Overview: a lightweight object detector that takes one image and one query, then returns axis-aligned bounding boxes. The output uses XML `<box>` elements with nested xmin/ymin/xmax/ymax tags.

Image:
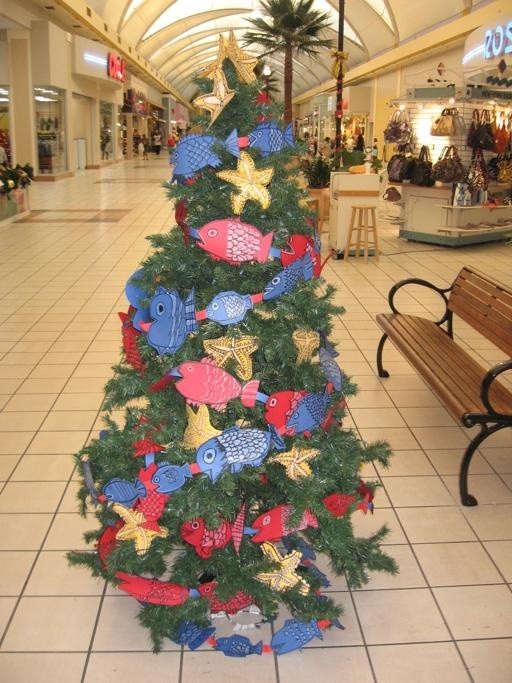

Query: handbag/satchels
<box><xmin>383</xmin><ymin>108</ymin><xmax>512</xmax><ymax>206</ymax></box>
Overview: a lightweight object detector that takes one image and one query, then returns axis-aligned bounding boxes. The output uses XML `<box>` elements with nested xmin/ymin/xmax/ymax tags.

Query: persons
<box><xmin>366</xmin><ymin>145</ymin><xmax>378</xmax><ymax>160</ymax></box>
<box><xmin>172</xmin><ymin>126</ymin><xmax>182</xmax><ymax>141</ymax></box>
<box><xmin>323</xmin><ymin>137</ymin><xmax>331</xmax><ymax>148</ymax></box>
<box><xmin>356</xmin><ymin>134</ymin><xmax>365</xmax><ymax>153</ymax></box>
<box><xmin>138</xmin><ymin>134</ymin><xmax>148</xmax><ymax>160</ymax></box>
<box><xmin>167</xmin><ymin>135</ymin><xmax>174</xmax><ymax>155</ymax></box>
<box><xmin>100</xmin><ymin>131</ymin><xmax>110</xmax><ymax>160</ymax></box>
<box><xmin>153</xmin><ymin>130</ymin><xmax>162</xmax><ymax>155</ymax></box>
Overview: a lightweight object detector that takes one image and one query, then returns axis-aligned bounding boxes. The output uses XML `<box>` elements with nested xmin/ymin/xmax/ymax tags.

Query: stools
<box><xmin>342</xmin><ymin>202</ymin><xmax>380</xmax><ymax>265</ymax></box>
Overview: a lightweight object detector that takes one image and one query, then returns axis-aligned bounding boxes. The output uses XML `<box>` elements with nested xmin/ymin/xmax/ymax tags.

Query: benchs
<box><xmin>374</xmin><ymin>265</ymin><xmax>512</xmax><ymax>507</ymax></box>
